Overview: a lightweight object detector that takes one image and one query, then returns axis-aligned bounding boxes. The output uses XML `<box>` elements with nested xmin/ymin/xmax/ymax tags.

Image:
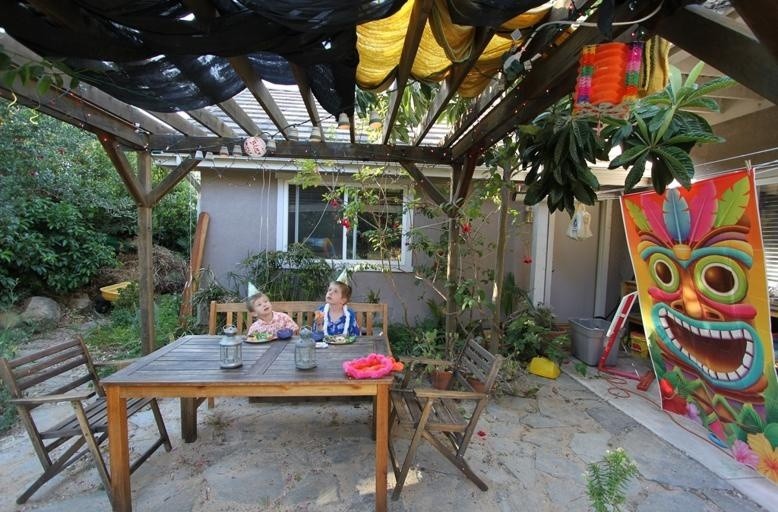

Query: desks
<box><xmin>98</xmin><ymin>335</ymin><xmax>393</xmax><ymax>511</ymax></box>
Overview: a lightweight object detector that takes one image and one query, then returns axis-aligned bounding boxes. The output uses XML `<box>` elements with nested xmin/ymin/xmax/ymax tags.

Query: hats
<box><xmin>335</xmin><ymin>267</ymin><xmax>348</xmax><ymax>286</ymax></box>
<box><xmin>247</xmin><ymin>282</ymin><xmax>259</xmax><ymax>297</ymax></box>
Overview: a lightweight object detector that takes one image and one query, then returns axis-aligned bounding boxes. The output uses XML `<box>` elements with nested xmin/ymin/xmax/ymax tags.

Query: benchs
<box><xmin>387</xmin><ymin>335</ymin><xmax>503</xmax><ymax>501</ymax></box>
<box><xmin>208</xmin><ymin>300</ymin><xmax>394</xmax><ymax>410</ymax></box>
<box><xmin>0</xmin><ymin>333</ymin><xmax>172</xmax><ymax>512</ymax></box>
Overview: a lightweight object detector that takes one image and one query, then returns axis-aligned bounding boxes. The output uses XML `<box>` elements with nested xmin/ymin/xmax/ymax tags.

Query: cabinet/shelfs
<box><xmin>619</xmin><ymin>280</ymin><xmax>643</xmax><ymax>337</ymax></box>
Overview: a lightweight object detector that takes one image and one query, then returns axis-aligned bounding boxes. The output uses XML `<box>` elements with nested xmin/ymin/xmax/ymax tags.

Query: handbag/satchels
<box><xmin>431</xmin><ymin>370</ymin><xmax>452</xmax><ymax>390</ymax></box>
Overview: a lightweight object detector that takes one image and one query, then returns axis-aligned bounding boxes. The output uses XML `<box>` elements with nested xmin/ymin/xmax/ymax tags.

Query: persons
<box><xmin>311</xmin><ymin>268</ymin><xmax>361</xmax><ymax>336</ymax></box>
<box><xmin>246</xmin><ymin>281</ymin><xmax>299</xmax><ymax>336</ymax></box>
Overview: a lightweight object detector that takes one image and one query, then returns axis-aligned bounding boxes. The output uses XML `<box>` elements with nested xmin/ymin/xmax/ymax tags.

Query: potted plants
<box><xmin>412</xmin><ymin>329</ymin><xmax>458</xmax><ymax>389</ymax></box>
<box><xmin>517</xmin><ymin>287</ymin><xmax>567</xmax><ymax>356</ymax></box>
<box><xmin>467</xmin><ymin>336</ymin><xmax>487</xmax><ymax>394</ymax></box>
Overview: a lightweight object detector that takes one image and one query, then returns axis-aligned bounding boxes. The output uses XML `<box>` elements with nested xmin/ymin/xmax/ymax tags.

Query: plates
<box><xmin>323</xmin><ymin>335</ymin><xmax>356</xmax><ymax>344</ymax></box>
<box><xmin>244</xmin><ymin>337</ymin><xmax>277</xmax><ymax>343</ymax></box>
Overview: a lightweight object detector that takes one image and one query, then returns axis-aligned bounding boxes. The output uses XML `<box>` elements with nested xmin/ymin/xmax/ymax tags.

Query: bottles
<box><xmin>294</xmin><ymin>325</ymin><xmax>316</xmax><ymax>369</ymax></box>
<box><xmin>219</xmin><ymin>324</ymin><xmax>242</xmax><ymax>369</ymax></box>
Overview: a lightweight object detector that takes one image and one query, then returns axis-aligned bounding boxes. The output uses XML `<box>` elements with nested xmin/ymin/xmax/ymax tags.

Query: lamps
<box><xmin>194</xmin><ymin>90</ymin><xmax>384</xmax><ymax>161</ymax></box>
